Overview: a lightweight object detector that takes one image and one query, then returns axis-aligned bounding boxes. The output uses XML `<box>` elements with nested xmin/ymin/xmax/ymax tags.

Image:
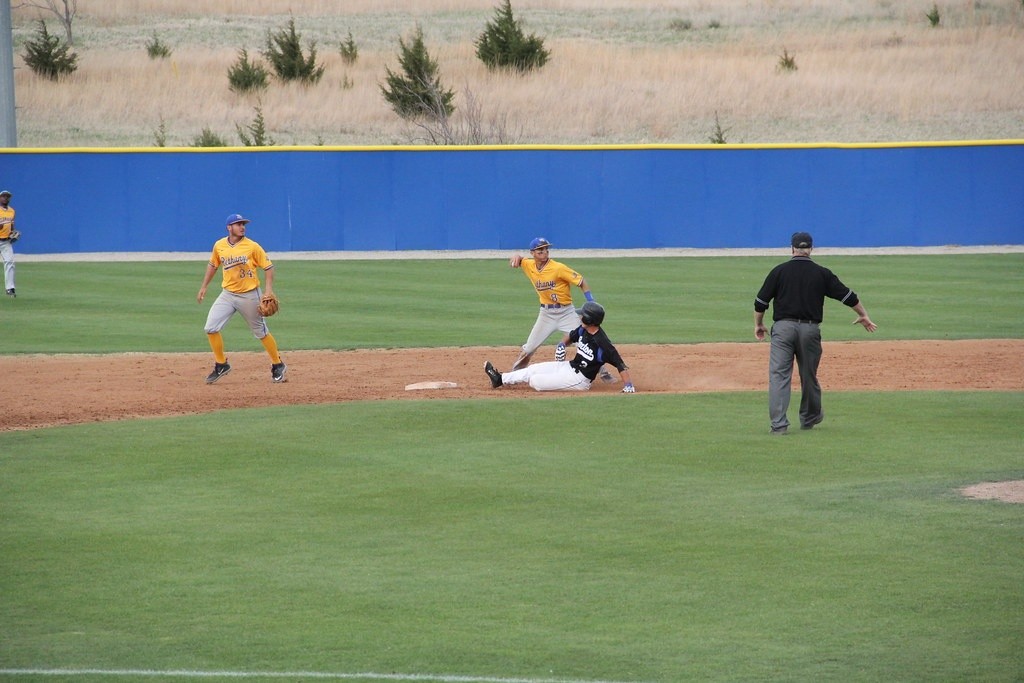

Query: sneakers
<box><xmin>601</xmin><ymin>371</ymin><xmax>617</xmax><ymax>383</ymax></box>
<box><xmin>205</xmin><ymin>357</ymin><xmax>231</xmax><ymax>384</ymax></box>
<box><xmin>6</xmin><ymin>288</ymin><xmax>16</xmax><ymax>298</ymax></box>
<box><xmin>484</xmin><ymin>360</ymin><xmax>502</xmax><ymax>389</ymax></box>
<box><xmin>271</xmin><ymin>357</ymin><xmax>287</xmax><ymax>383</ymax></box>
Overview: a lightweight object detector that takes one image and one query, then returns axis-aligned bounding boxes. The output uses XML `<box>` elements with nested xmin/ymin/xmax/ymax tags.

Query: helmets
<box><xmin>573</xmin><ymin>302</ymin><xmax>605</xmax><ymax>325</ymax></box>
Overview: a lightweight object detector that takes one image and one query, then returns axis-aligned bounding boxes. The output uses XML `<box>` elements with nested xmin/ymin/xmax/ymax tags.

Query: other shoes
<box><xmin>769</xmin><ymin>428</ymin><xmax>787</xmax><ymax>436</ymax></box>
<box><xmin>800</xmin><ymin>409</ymin><xmax>824</xmax><ymax>429</ymax></box>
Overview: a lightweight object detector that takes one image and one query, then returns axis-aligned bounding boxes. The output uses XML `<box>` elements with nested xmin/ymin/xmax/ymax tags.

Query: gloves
<box><xmin>555</xmin><ymin>343</ymin><xmax>566</xmax><ymax>362</ymax></box>
<box><xmin>620</xmin><ymin>383</ymin><xmax>635</xmax><ymax>394</ymax></box>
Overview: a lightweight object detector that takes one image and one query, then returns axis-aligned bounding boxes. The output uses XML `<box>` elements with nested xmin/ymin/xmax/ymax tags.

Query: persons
<box><xmin>509</xmin><ymin>237</ymin><xmax>619</xmax><ymax>384</ymax></box>
<box><xmin>753</xmin><ymin>231</ymin><xmax>878</xmax><ymax>435</ymax></box>
<box><xmin>483</xmin><ymin>301</ymin><xmax>635</xmax><ymax>393</ymax></box>
<box><xmin>197</xmin><ymin>214</ymin><xmax>287</xmax><ymax>385</ymax></box>
<box><xmin>0</xmin><ymin>190</ymin><xmax>21</xmax><ymax>298</ymax></box>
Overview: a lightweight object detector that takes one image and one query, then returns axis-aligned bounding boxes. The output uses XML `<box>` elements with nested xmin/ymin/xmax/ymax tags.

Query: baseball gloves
<box><xmin>257</xmin><ymin>293</ymin><xmax>279</xmax><ymax>317</ymax></box>
<box><xmin>9</xmin><ymin>231</ymin><xmax>21</xmax><ymax>244</ymax></box>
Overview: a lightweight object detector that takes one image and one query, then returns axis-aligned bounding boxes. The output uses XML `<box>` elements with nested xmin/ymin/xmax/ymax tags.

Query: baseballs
<box><xmin>512</xmin><ymin>261</ymin><xmax>515</xmax><ymax>267</ymax></box>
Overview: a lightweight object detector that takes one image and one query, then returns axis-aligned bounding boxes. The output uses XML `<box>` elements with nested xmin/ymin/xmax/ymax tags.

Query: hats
<box><xmin>226</xmin><ymin>214</ymin><xmax>250</xmax><ymax>225</ymax></box>
<box><xmin>529</xmin><ymin>238</ymin><xmax>553</xmax><ymax>250</ymax></box>
<box><xmin>0</xmin><ymin>190</ymin><xmax>12</xmax><ymax>196</ymax></box>
<box><xmin>792</xmin><ymin>231</ymin><xmax>813</xmax><ymax>249</ymax></box>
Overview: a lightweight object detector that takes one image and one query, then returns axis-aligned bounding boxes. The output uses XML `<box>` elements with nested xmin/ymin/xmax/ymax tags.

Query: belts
<box><xmin>779</xmin><ymin>318</ymin><xmax>816</xmax><ymax>324</ymax></box>
<box><xmin>541</xmin><ymin>304</ymin><xmax>565</xmax><ymax>308</ymax></box>
<box><xmin>570</xmin><ymin>364</ymin><xmax>593</xmax><ymax>384</ymax></box>
